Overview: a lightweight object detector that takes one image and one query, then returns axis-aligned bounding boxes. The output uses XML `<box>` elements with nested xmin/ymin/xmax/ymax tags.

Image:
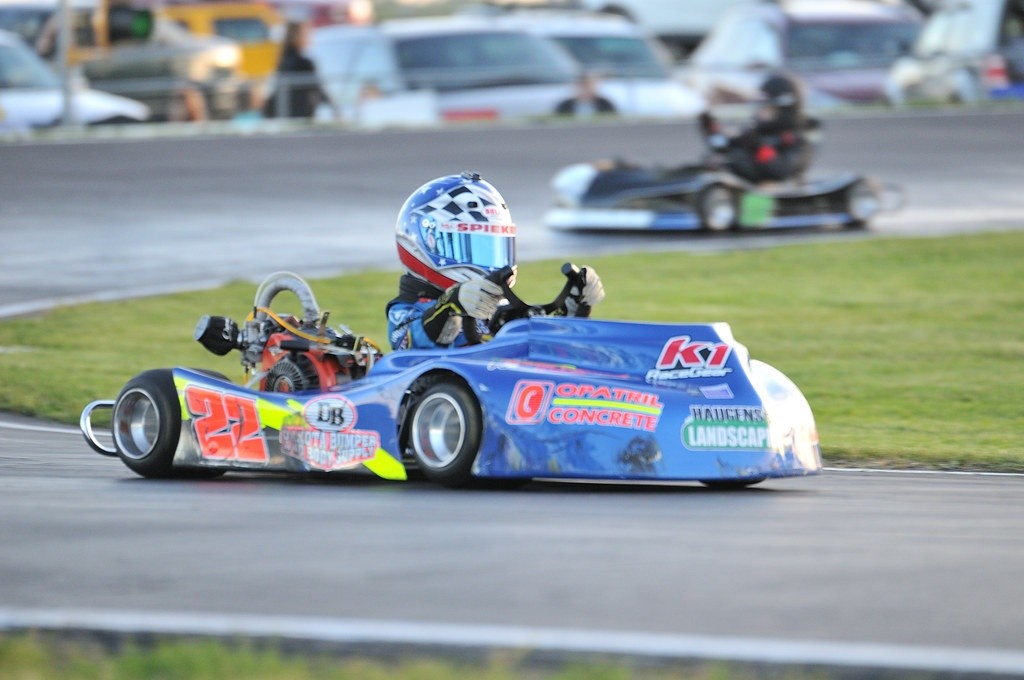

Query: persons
<box><xmin>274</xmin><ymin>22</ymin><xmax>336</xmax><ymax>118</ymax></box>
<box><xmin>386</xmin><ymin>171</ymin><xmax>604</xmax><ymax>350</ymax></box>
<box><xmin>554</xmin><ymin>69</ymin><xmax>618</xmax><ymax>116</ymax></box>
<box><xmin>160</xmin><ymin>84</ymin><xmax>204</xmax><ymax>121</ymax></box>
<box><xmin>699</xmin><ymin>77</ymin><xmax>814</xmax><ymax>183</ymax></box>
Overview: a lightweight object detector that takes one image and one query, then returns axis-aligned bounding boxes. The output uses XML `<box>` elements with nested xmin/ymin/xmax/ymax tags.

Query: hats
<box><xmin>757</xmin><ymin>76</ymin><xmax>802</xmax><ymax>131</ymax></box>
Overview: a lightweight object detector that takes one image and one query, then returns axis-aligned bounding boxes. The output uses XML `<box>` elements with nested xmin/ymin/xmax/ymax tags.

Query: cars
<box><xmin>0</xmin><ymin>0</ymin><xmax>375</xmax><ymax>132</ymax></box>
<box><xmin>684</xmin><ymin>2</ymin><xmax>930</xmax><ymax>104</ymax></box>
<box><xmin>285</xmin><ymin>0</ymin><xmax>756</xmax><ymax>124</ymax></box>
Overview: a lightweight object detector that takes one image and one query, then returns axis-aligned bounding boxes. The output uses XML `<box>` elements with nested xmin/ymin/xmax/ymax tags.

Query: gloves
<box><xmin>554</xmin><ymin>265</ymin><xmax>605</xmax><ymax>319</ymax></box>
<box><xmin>447</xmin><ymin>277</ymin><xmax>503</xmax><ymax>320</ymax></box>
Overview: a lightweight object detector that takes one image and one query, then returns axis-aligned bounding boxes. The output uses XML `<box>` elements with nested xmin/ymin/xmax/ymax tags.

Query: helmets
<box><xmin>394</xmin><ymin>170</ymin><xmax>518</xmax><ymax>294</ymax></box>
